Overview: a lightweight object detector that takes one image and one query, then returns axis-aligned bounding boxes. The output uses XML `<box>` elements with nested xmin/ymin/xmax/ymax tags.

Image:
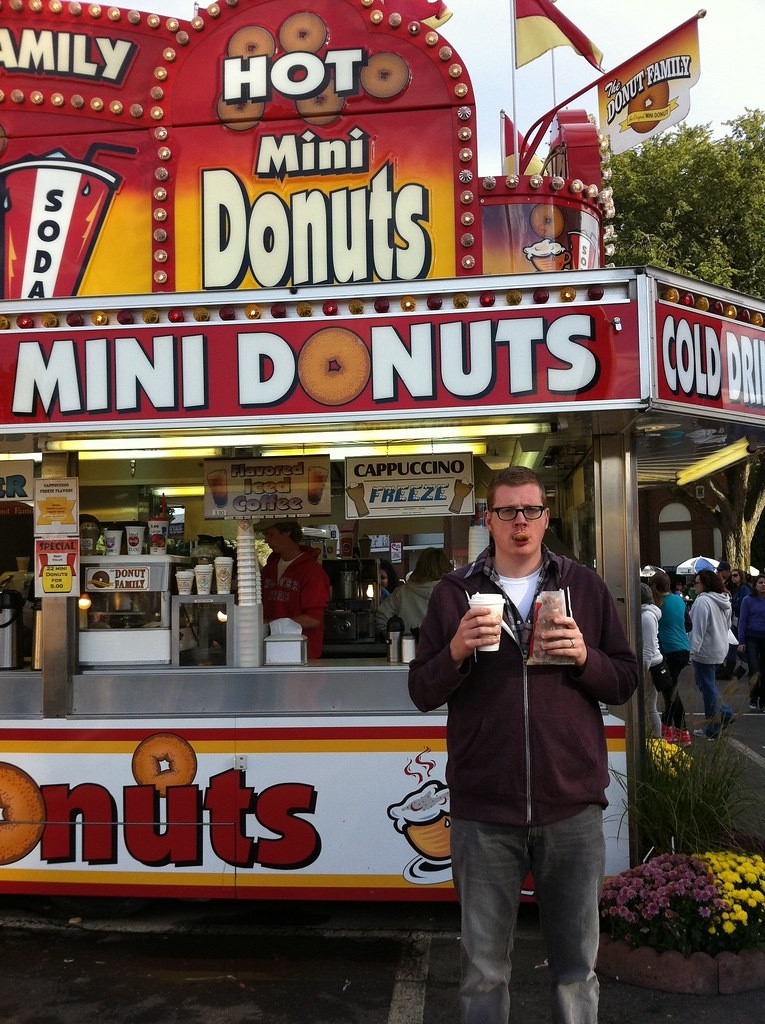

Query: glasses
<box><xmin>731</xmin><ymin>574</ymin><xmax>738</xmax><ymax>578</ymax></box>
<box><xmin>694</xmin><ymin>582</ymin><xmax>701</xmax><ymax>584</ymax></box>
<box><xmin>490</xmin><ymin>505</ymin><xmax>546</xmax><ymax>520</ymax></box>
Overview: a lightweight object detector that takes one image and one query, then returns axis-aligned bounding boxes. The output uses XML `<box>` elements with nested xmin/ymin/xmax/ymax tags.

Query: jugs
<box><xmin>31</xmin><ymin>599</ymin><xmax>42</xmax><ymax>670</ymax></box>
<box><xmin>0</xmin><ymin>575</ymin><xmax>26</xmax><ymax>669</ymax></box>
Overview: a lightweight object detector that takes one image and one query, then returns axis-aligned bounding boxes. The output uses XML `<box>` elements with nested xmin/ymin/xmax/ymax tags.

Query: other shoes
<box><xmin>693</xmin><ymin>728</ymin><xmax>705</xmax><ymax>737</ymax></box>
<box><xmin>748</xmin><ymin>699</ymin><xmax>758</xmax><ymax>709</ymax></box>
<box><xmin>761</xmin><ymin>705</ymin><xmax>765</xmax><ymax>712</ymax></box>
<box><xmin>721</xmin><ymin>718</ymin><xmax>735</xmax><ymax>729</ymax></box>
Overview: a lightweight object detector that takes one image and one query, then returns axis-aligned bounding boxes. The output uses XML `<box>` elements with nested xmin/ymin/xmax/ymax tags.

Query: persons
<box><xmin>672</xmin><ymin>583</ymin><xmax>685</xmax><ymax>598</ymax></box>
<box><xmin>716</xmin><ymin>562</ymin><xmax>765</xmax><ymax>710</ymax></box>
<box><xmin>376</xmin><ymin>548</ymin><xmax>453</xmax><ymax>639</ymax></box>
<box><xmin>690</xmin><ymin>570</ymin><xmax>735</xmax><ymax>740</ymax></box>
<box><xmin>379</xmin><ymin>559</ymin><xmax>398</xmax><ymax>601</ymax></box>
<box><xmin>253</xmin><ymin>517</ymin><xmax>329</xmax><ymax>659</ymax></box>
<box><xmin>407</xmin><ymin>466</ymin><xmax>639</xmax><ymax>1024</ymax></box>
<box><xmin>649</xmin><ymin>574</ymin><xmax>691</xmax><ymax>747</ymax></box>
<box><xmin>640</xmin><ymin>584</ymin><xmax>662</xmax><ymax>741</ymax></box>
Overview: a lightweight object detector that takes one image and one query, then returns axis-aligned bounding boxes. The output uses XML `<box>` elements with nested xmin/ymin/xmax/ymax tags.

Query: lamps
<box><xmin>149</xmin><ymin>485</ymin><xmax>205</xmax><ymax>500</ymax></box>
<box><xmin>252</xmin><ymin>441</ymin><xmax>500</xmax><ymax>467</ymax></box>
<box><xmin>675</xmin><ymin>435</ymin><xmax>763</xmax><ymax>489</ymax></box>
<box><xmin>34</xmin><ymin>411</ymin><xmax>572</xmax><ymax>454</ymax></box>
<box><xmin>74</xmin><ymin>446</ymin><xmax>226</xmax><ymax>462</ymax></box>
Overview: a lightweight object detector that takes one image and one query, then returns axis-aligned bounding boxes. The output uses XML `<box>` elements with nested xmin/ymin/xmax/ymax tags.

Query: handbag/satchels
<box><xmin>732</xmin><ymin>616</ymin><xmax>738</xmax><ymax>627</ymax></box>
<box><xmin>650</xmin><ymin>659</ymin><xmax>671</xmax><ymax>692</ymax></box>
<box><xmin>684</xmin><ymin>611</ymin><xmax>692</xmax><ymax>632</ymax></box>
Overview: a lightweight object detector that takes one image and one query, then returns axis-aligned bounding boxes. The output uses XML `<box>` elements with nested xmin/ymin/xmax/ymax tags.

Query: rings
<box><xmin>570</xmin><ymin>639</ymin><xmax>575</xmax><ymax>649</ymax></box>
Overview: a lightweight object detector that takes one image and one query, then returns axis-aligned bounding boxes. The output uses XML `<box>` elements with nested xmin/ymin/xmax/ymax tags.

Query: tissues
<box><xmin>262</xmin><ymin>616</ymin><xmax>309</xmax><ymax>666</ymax></box>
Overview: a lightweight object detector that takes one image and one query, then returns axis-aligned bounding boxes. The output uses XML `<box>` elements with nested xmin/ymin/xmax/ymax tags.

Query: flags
<box><xmin>510</xmin><ymin>0</ymin><xmax>604</xmax><ymax>73</ymax></box>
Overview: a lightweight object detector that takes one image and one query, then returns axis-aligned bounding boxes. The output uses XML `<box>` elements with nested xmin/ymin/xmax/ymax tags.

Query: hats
<box><xmin>717</xmin><ymin>563</ymin><xmax>730</xmax><ymax>571</ymax></box>
<box><xmin>254</xmin><ymin>518</ymin><xmax>297</xmax><ymax>530</ymax></box>
<box><xmin>641</xmin><ymin>565</ymin><xmax>657</xmax><ymax>577</ymax></box>
<box><xmin>640</xmin><ymin>583</ymin><xmax>656</xmax><ymax>603</ymax></box>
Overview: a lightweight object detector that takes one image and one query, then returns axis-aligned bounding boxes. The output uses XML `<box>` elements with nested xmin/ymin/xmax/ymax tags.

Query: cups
<box><xmin>468</xmin><ymin>497</ymin><xmax>491</xmax><ymax>565</ymax></box>
<box><xmin>358</xmin><ymin>538</ymin><xmax>372</xmax><ymax>558</ymax></box>
<box><xmin>194</xmin><ymin>564</ymin><xmax>214</xmax><ymax>595</ymax></box>
<box><xmin>307</xmin><ymin>466</ymin><xmax>329</xmax><ymax>505</ymax></box>
<box><xmin>236</xmin><ymin>519</ymin><xmax>263</xmax><ymax>667</ymax></box>
<box><xmin>16</xmin><ymin>556</ymin><xmax>31</xmax><ymax>573</ymax></box>
<box><xmin>147</xmin><ymin>518</ymin><xmax>170</xmax><ymax>554</ymax></box>
<box><xmin>213</xmin><ymin>556</ymin><xmax>234</xmax><ymax>595</ymax></box>
<box><xmin>125</xmin><ymin>526</ymin><xmax>146</xmax><ymax>555</ymax></box>
<box><xmin>79</xmin><ymin>522</ymin><xmax>100</xmax><ymax>556</ymax></box>
<box><xmin>324</xmin><ymin>539</ymin><xmax>338</xmax><ymax>560</ymax></box>
<box><xmin>102</xmin><ymin>530</ymin><xmax>123</xmax><ymax>555</ymax></box>
<box><xmin>338</xmin><ymin>532</ymin><xmax>354</xmax><ymax>559</ymax></box>
<box><xmin>468</xmin><ymin>594</ymin><xmax>504</xmax><ymax>651</ymax></box>
<box><xmin>206</xmin><ymin>469</ymin><xmax>228</xmax><ymax>508</ymax></box>
<box><xmin>310</xmin><ymin>541</ymin><xmax>323</xmax><ymax>565</ymax></box>
<box><xmin>175</xmin><ymin>570</ymin><xmax>196</xmax><ymax>596</ymax></box>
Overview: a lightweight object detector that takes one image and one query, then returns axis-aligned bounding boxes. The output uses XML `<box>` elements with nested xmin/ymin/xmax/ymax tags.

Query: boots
<box><xmin>674</xmin><ymin>729</ymin><xmax>691</xmax><ymax>746</ymax></box>
<box><xmin>716</xmin><ymin>660</ymin><xmax>736</xmax><ymax>680</ymax></box>
<box><xmin>661</xmin><ymin>724</ymin><xmax>674</xmax><ymax>743</ymax></box>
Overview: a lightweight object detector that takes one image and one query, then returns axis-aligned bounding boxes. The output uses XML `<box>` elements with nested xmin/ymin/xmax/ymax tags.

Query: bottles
<box><xmin>386</xmin><ymin>612</ymin><xmax>405</xmax><ymax>663</ymax></box>
<box><xmin>401</xmin><ymin>635</ymin><xmax>416</xmax><ymax>664</ymax></box>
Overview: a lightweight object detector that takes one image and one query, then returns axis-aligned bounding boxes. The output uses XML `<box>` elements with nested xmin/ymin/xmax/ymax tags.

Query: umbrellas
<box><xmin>676</xmin><ymin>556</ymin><xmax>720</xmax><ymax>574</ymax></box>
<box><xmin>640</xmin><ymin>565</ymin><xmax>666</xmax><ymax>583</ymax></box>
